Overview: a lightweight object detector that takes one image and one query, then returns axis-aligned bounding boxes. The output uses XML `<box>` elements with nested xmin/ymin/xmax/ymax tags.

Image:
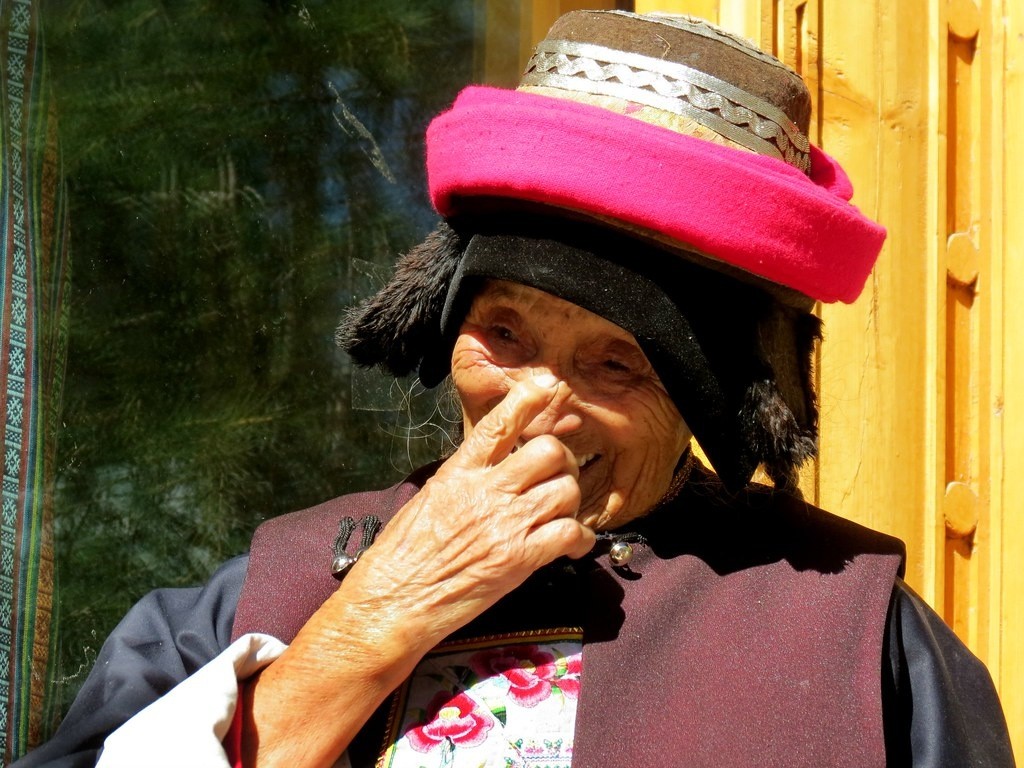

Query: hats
<box><xmin>428</xmin><ymin>10</ymin><xmax>889</xmax><ymax>305</ymax></box>
<box><xmin>328</xmin><ymin>197</ymin><xmax>819</xmax><ymax>497</ymax></box>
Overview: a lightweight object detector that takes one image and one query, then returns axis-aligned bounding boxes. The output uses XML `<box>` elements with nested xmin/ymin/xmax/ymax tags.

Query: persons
<box><xmin>19</xmin><ymin>9</ymin><xmax>1017</xmax><ymax>768</ymax></box>
